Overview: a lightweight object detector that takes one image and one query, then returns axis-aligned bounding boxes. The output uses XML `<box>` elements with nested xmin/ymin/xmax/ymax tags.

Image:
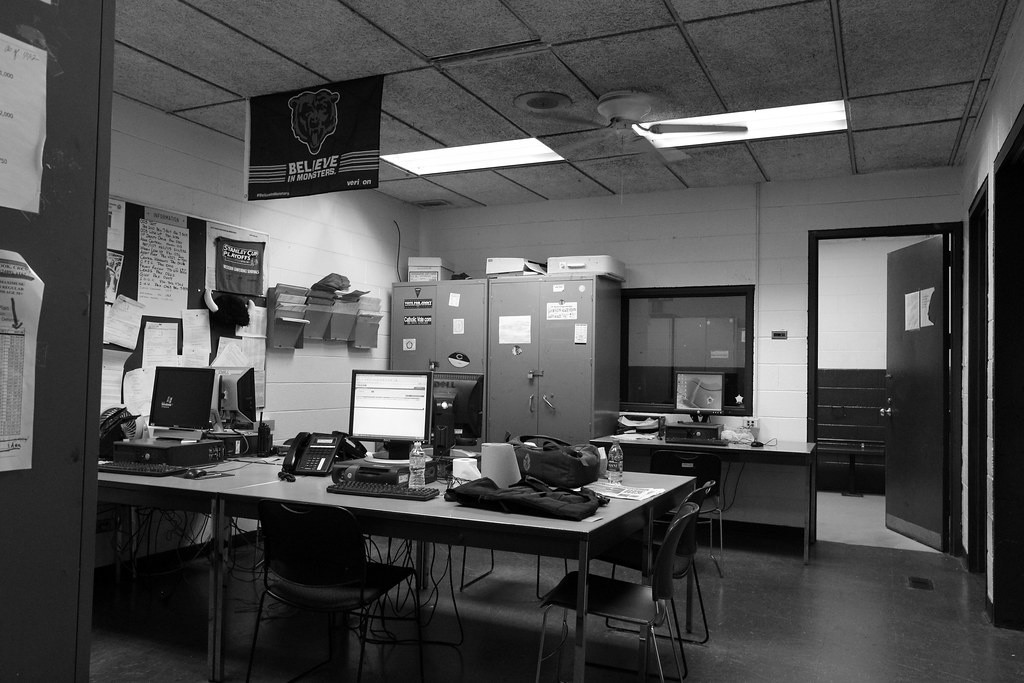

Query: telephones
<box><xmin>281</xmin><ymin>432</ymin><xmax>343</xmax><ymax>476</ymax></box>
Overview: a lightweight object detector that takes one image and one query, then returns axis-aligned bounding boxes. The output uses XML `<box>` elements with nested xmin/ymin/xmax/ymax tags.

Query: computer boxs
<box><xmin>114</xmin><ymin>433</ymin><xmax>272</xmax><ymax>467</ymax></box>
<box><xmin>330</xmin><ymin>452</ymin><xmax>482</xmax><ymax>488</ymax></box>
<box><xmin>664</xmin><ymin>422</ymin><xmax>724</xmax><ymax>439</ymax></box>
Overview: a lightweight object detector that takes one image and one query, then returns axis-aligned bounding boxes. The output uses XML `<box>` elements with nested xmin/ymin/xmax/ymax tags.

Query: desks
<box><xmin>590</xmin><ymin>435</ymin><xmax>817</xmax><ymax>563</ymax></box>
<box><xmin>96</xmin><ymin>455</ymin><xmax>697</xmax><ymax>683</ymax></box>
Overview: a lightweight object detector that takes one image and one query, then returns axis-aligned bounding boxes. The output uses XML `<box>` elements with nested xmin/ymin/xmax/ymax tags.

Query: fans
<box><xmin>534</xmin><ymin>90</ymin><xmax>745</xmax><ymax>167</ymax></box>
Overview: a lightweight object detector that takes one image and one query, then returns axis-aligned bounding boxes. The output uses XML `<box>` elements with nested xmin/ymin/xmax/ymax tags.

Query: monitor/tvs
<box><xmin>348</xmin><ymin>371</ymin><xmax>485</xmax><ymax>464</ymax></box>
<box><xmin>148</xmin><ymin>366</ymin><xmax>256</xmax><ymax>435</ymax></box>
<box><xmin>676</xmin><ymin>371</ymin><xmax>725</xmax><ymax>427</ymax></box>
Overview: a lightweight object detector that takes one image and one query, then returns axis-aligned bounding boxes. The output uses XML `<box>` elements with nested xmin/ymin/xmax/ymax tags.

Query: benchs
<box><xmin>817</xmin><ymin>437</ymin><xmax>885</xmax><ymax>496</ymax></box>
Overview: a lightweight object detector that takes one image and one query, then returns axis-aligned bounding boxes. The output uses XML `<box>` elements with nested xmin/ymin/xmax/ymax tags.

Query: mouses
<box><xmin>444</xmin><ymin>492</ymin><xmax>457</xmax><ymax>502</ymax></box>
<box><xmin>751</xmin><ymin>442</ymin><xmax>765</xmax><ymax>447</ymax></box>
<box><xmin>184</xmin><ymin>468</ymin><xmax>206</xmax><ymax>479</ymax></box>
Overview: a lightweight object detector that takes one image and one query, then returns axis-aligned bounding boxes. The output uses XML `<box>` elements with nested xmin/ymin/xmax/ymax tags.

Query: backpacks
<box><xmin>504</xmin><ymin>431</ymin><xmax>601</xmax><ymax>488</ymax></box>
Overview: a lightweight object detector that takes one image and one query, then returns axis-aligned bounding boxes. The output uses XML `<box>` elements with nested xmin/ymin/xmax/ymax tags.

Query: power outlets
<box><xmin>743</xmin><ymin>417</ymin><xmax>759</xmax><ymax>429</ymax></box>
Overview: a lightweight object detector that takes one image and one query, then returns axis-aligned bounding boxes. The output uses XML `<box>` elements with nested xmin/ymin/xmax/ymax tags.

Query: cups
<box><xmin>599</xmin><ymin>457</ymin><xmax>606</xmax><ymax>477</ymax></box>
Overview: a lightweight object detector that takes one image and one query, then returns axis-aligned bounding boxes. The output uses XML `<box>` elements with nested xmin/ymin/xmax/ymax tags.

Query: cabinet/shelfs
<box><xmin>391</xmin><ymin>280</ymin><xmax>489</xmax><ymax>453</ymax></box>
<box><xmin>487</xmin><ymin>278</ymin><xmax>622</xmax><ymax>448</ymax></box>
<box><xmin>644</xmin><ymin>315</ymin><xmax>737</xmax><ymax>403</ymax></box>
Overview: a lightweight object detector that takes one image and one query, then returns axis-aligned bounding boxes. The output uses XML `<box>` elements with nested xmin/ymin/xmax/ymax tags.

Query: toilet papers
<box><xmin>480</xmin><ymin>442</ymin><xmax>522</xmax><ymax>488</ymax></box>
<box><xmin>451</xmin><ymin>457</ymin><xmax>481</xmax><ymax>489</ymax></box>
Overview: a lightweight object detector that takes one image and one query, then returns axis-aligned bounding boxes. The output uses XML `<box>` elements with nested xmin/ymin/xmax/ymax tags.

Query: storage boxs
<box><xmin>407</xmin><ymin>256</ymin><xmax>456</xmax><ymax>281</ymax></box>
<box><xmin>485</xmin><ymin>258</ymin><xmax>530</xmax><ymax>278</ymax></box>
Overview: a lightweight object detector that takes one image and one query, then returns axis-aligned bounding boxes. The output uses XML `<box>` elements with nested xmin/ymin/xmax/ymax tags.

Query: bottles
<box><xmin>608</xmin><ymin>439</ymin><xmax>623</xmax><ymax>485</ymax></box>
<box><xmin>408</xmin><ymin>442</ymin><xmax>426</xmax><ymax>491</ymax></box>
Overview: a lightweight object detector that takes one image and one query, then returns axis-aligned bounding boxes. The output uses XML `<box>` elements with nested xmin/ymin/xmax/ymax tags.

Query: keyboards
<box><xmin>326</xmin><ymin>481</ymin><xmax>440</xmax><ymax>501</ymax></box>
<box><xmin>666</xmin><ymin>437</ymin><xmax>730</xmax><ymax>447</ymax></box>
<box><xmin>98</xmin><ymin>461</ymin><xmax>191</xmax><ymax>478</ymax></box>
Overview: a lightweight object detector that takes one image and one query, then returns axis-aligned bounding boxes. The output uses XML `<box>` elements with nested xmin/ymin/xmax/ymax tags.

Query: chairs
<box><xmin>246</xmin><ymin>499</ymin><xmax>423</xmax><ymax>683</ymax></box>
<box><xmin>648</xmin><ymin>448</ymin><xmax>725</xmax><ymax>577</ymax></box>
<box><xmin>460</xmin><ymin>435</ymin><xmax>573</xmax><ymax>598</ymax></box>
<box><xmin>535</xmin><ymin>503</ymin><xmax>700</xmax><ymax>683</ymax></box>
<box><xmin>597</xmin><ymin>479</ymin><xmax>715</xmax><ymax>642</ymax></box>
